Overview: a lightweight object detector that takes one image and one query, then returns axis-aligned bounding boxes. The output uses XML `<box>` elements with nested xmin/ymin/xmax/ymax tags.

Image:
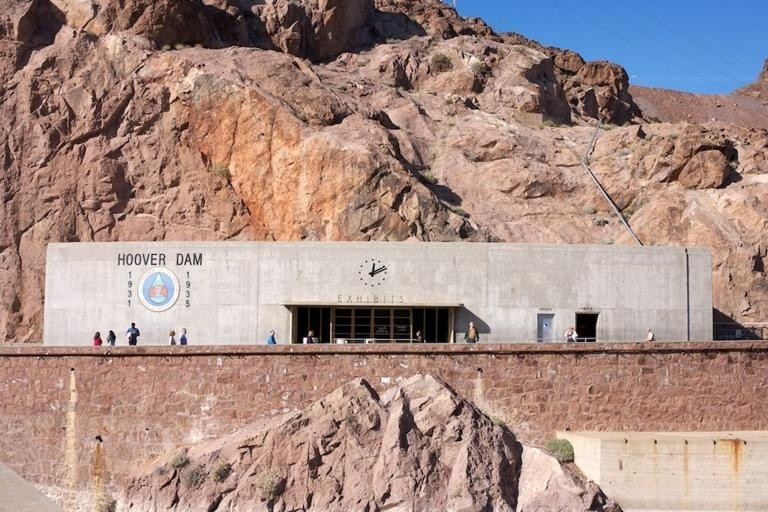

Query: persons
<box><xmin>642</xmin><ymin>328</ymin><xmax>655</xmax><ymax>341</ymax></box>
<box><xmin>416</xmin><ymin>331</ymin><xmax>424</xmax><ymax>343</ymax></box>
<box><xmin>168</xmin><ymin>330</ymin><xmax>176</xmax><ymax>346</ymax></box>
<box><xmin>267</xmin><ymin>330</ymin><xmax>276</xmax><ymax>344</ymax></box>
<box><xmin>178</xmin><ymin>328</ymin><xmax>187</xmax><ymax>345</ymax></box>
<box><xmin>107</xmin><ymin>330</ymin><xmax>116</xmax><ymax>346</ymax></box>
<box><xmin>126</xmin><ymin>322</ymin><xmax>140</xmax><ymax>345</ymax></box>
<box><xmin>564</xmin><ymin>326</ymin><xmax>578</xmax><ymax>342</ymax></box>
<box><xmin>94</xmin><ymin>331</ymin><xmax>103</xmax><ymax>345</ymax></box>
<box><xmin>306</xmin><ymin>330</ymin><xmax>314</xmax><ymax>343</ymax></box>
<box><xmin>463</xmin><ymin>320</ymin><xmax>479</xmax><ymax>343</ymax></box>
